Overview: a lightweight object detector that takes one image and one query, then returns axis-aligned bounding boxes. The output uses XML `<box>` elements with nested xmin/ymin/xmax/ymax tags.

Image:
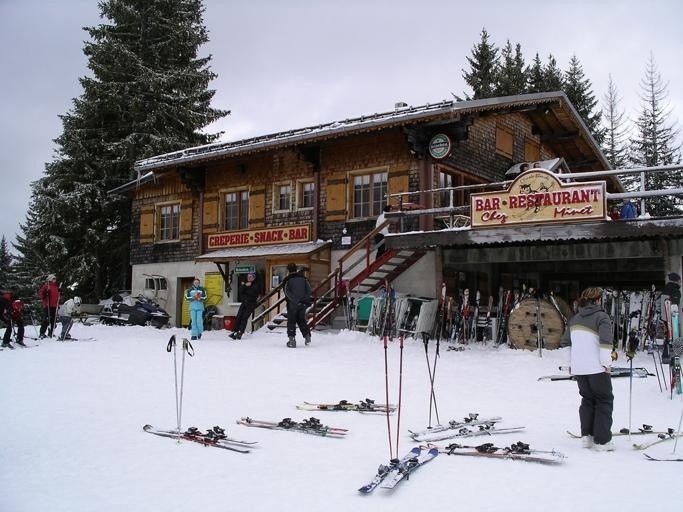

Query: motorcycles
<box><xmin>98</xmin><ymin>293</ymin><xmax>171</xmax><ymax>329</ymax></box>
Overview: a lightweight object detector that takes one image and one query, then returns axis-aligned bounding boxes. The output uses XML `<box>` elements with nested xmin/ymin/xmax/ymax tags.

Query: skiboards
<box><xmin>644</xmin><ymin>453</ymin><xmax>683</xmax><ymax>462</ymax></box>
<box><xmin>296</xmin><ymin>398</ymin><xmax>398</xmax><ymax>415</ymax></box>
<box><xmin>357</xmin><ymin>448</ymin><xmax>438</xmax><ymax>493</ymax></box>
<box><xmin>236</xmin><ymin>417</ymin><xmax>348</xmax><ymax>438</ymax></box>
<box><xmin>604</xmin><ymin>287</ymin><xmax>682</xmax><ymax>400</ymax></box>
<box><xmin>143</xmin><ymin>425</ymin><xmax>258</xmax><ymax>453</ymax></box>
<box><xmin>538</xmin><ymin>366</ymin><xmax>655</xmax><ymax>382</ymax></box>
<box><xmin>341</xmin><ymin>279</ymin><xmax>568</xmax><ymax>357</ymax></box>
<box><xmin>0</xmin><ymin>334</ymin><xmax>96</xmax><ymax>351</ymax></box>
<box><xmin>409</xmin><ymin>414</ymin><xmax>526</xmax><ymax>443</ymax></box>
<box><xmin>419</xmin><ymin>442</ymin><xmax>566</xmax><ymax>464</ymax></box>
<box><xmin>566</xmin><ymin>425</ymin><xmax>683</xmax><ymax>450</ymax></box>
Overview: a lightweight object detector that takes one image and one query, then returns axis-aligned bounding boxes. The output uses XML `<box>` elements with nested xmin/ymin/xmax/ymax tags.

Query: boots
<box><xmin>305</xmin><ymin>332</ymin><xmax>311</xmax><ymax>345</ymax></box>
<box><xmin>287</xmin><ymin>335</ymin><xmax>295</xmax><ymax>347</ymax></box>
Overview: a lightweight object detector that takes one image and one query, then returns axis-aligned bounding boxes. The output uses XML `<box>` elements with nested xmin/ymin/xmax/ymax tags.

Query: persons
<box><xmin>569</xmin><ymin>286</ymin><xmax>617</xmax><ymax>452</ymax></box>
<box><xmin>185</xmin><ymin>278</ymin><xmax>207</xmax><ymax>340</ymax></box>
<box><xmin>621</xmin><ymin>198</ymin><xmax>638</xmax><ymax>218</ymax></box>
<box><xmin>57</xmin><ymin>295</ymin><xmax>82</xmax><ymax>341</ymax></box>
<box><xmin>610</xmin><ymin>206</ymin><xmax>619</xmax><ymax>218</ymax></box>
<box><xmin>533</xmin><ymin>163</ymin><xmax>542</xmax><ymax>169</ymax></box>
<box><xmin>374</xmin><ymin>205</ymin><xmax>393</xmax><ymax>259</ymax></box>
<box><xmin>0</xmin><ymin>290</ymin><xmax>25</xmax><ymax>346</ymax></box>
<box><xmin>520</xmin><ymin>163</ymin><xmax>530</xmax><ymax>171</ymax></box>
<box><xmin>38</xmin><ymin>274</ymin><xmax>60</xmax><ymax>338</ymax></box>
<box><xmin>228</xmin><ymin>271</ymin><xmax>260</xmax><ymax>339</ymax></box>
<box><xmin>282</xmin><ymin>262</ymin><xmax>314</xmax><ymax>348</ymax></box>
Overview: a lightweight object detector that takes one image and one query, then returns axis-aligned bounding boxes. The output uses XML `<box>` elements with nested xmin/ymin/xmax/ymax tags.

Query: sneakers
<box><xmin>191</xmin><ymin>333</ymin><xmax>201</xmax><ymax>340</ymax></box>
<box><xmin>228</xmin><ymin>330</ymin><xmax>236</xmax><ymax>340</ymax></box>
<box><xmin>582</xmin><ymin>434</ymin><xmax>593</xmax><ymax>448</ymax></box>
<box><xmin>233</xmin><ymin>330</ymin><xmax>242</xmax><ymax>340</ymax></box>
<box><xmin>591</xmin><ymin>440</ymin><xmax>618</xmax><ymax>451</ymax></box>
<box><xmin>2</xmin><ymin>333</ymin><xmax>70</xmax><ymax>348</ymax></box>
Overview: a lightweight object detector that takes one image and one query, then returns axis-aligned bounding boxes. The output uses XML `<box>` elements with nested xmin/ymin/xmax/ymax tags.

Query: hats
<box><xmin>46</xmin><ymin>274</ymin><xmax>56</xmax><ymax>282</ymax></box>
<box><xmin>73</xmin><ymin>296</ymin><xmax>80</xmax><ymax>304</ymax></box>
